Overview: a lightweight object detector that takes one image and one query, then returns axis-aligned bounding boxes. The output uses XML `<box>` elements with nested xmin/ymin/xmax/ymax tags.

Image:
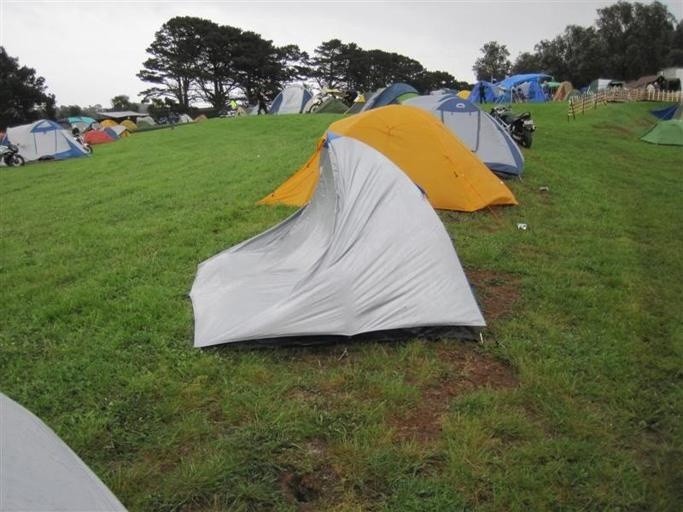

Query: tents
<box><xmin>0</xmin><ymin>109</ymin><xmax>208</xmax><ymax>165</ymax></box>
<box><xmin>640</xmin><ymin>102</ymin><xmax>683</xmax><ymax>146</ymax></box>
<box><xmin>255</xmin><ymin>81</ymin><xmax>525</xmax><ymax>214</ymax></box>
<box><xmin>456</xmin><ymin>73</ymin><xmax>624</xmax><ymax>107</ymax></box>
<box><xmin>0</xmin><ymin>393</ymin><xmax>128</xmax><ymax>512</ymax></box>
<box><xmin>187</xmin><ymin>131</ymin><xmax>487</xmax><ymax>349</ymax></box>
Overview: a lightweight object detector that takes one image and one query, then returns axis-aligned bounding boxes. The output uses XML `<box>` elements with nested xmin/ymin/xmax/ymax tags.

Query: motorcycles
<box><xmin>488</xmin><ymin>104</ymin><xmax>537</xmax><ymax>148</ymax></box>
<box><xmin>71</xmin><ymin>127</ymin><xmax>92</xmax><ymax>155</ymax></box>
<box><xmin>0</xmin><ymin>143</ymin><xmax>25</xmax><ymax>167</ymax></box>
